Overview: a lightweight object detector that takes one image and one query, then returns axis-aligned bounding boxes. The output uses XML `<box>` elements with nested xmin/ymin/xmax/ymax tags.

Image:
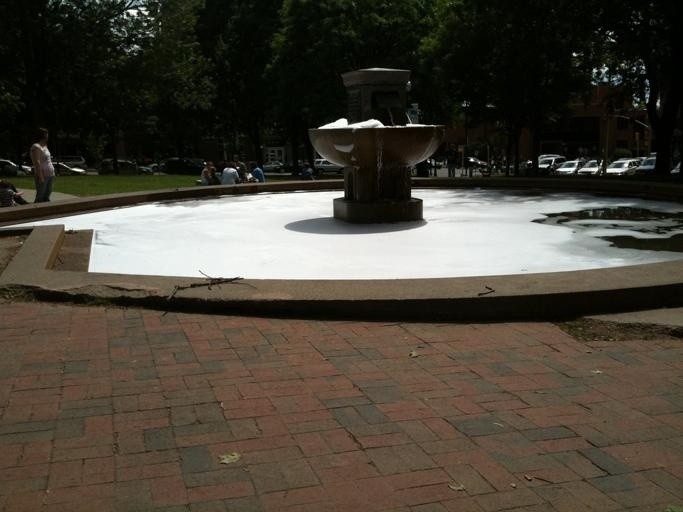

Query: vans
<box><xmin>313</xmin><ymin>158</ymin><xmax>344</xmax><ymax>174</ymax></box>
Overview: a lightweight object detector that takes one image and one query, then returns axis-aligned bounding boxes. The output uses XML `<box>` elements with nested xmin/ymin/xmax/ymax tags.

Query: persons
<box><xmin>417</xmin><ymin>160</ymin><xmax>433</xmax><ymax>177</ymax></box>
<box><xmin>198</xmin><ymin>154</ymin><xmax>268</xmax><ymax>186</ymax></box>
<box><xmin>443</xmin><ymin>143</ymin><xmax>459</xmax><ymax>177</ymax></box>
<box><xmin>303</xmin><ymin>162</ymin><xmax>315</xmax><ymax>180</ymax></box>
<box><xmin>467</xmin><ymin>147</ymin><xmax>607</xmax><ymax>177</ymax></box>
<box><xmin>0</xmin><ymin>180</ymin><xmax>27</xmax><ymax>208</ymax></box>
<box><xmin>28</xmin><ymin>127</ymin><xmax>55</xmax><ymax>204</ymax></box>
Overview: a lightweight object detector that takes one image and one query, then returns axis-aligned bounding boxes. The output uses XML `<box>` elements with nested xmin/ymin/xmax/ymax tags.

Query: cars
<box><xmin>0</xmin><ymin>153</ymin><xmax>289</xmax><ymax>178</ymax></box>
<box><xmin>424</xmin><ymin>150</ymin><xmax>681</xmax><ymax>177</ymax></box>
